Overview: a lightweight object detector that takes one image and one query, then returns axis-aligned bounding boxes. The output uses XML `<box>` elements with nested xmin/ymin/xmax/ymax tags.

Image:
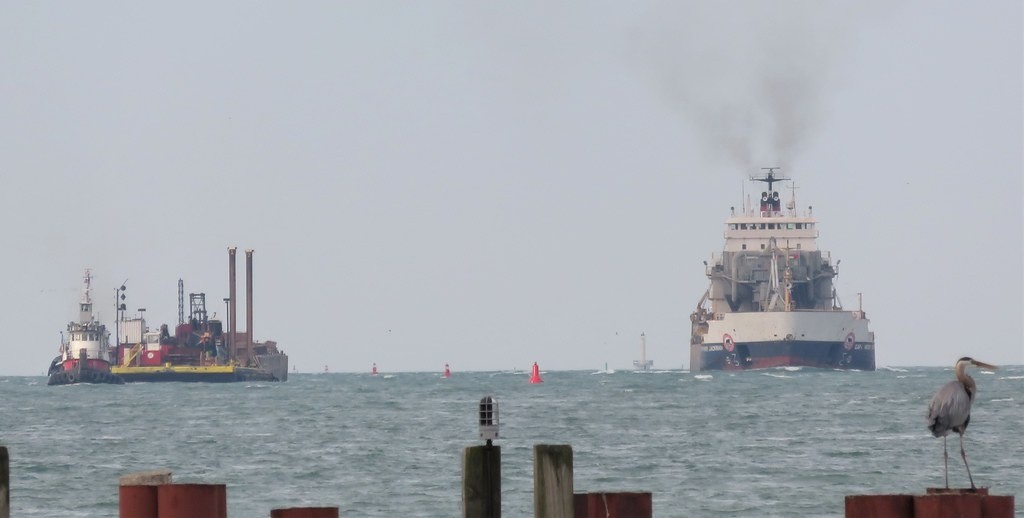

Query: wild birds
<box><xmin>926</xmin><ymin>357</ymin><xmax>999</xmax><ymax>488</ymax></box>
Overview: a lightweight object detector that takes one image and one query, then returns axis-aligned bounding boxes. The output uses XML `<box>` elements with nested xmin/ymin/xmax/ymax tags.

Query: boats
<box><xmin>107</xmin><ymin>247</ymin><xmax>289</xmax><ymax>382</ymax></box>
<box><xmin>689</xmin><ymin>164</ymin><xmax>876</xmax><ymax>376</ymax></box>
<box><xmin>47</xmin><ymin>268</ymin><xmax>125</xmax><ymax>386</ymax></box>
